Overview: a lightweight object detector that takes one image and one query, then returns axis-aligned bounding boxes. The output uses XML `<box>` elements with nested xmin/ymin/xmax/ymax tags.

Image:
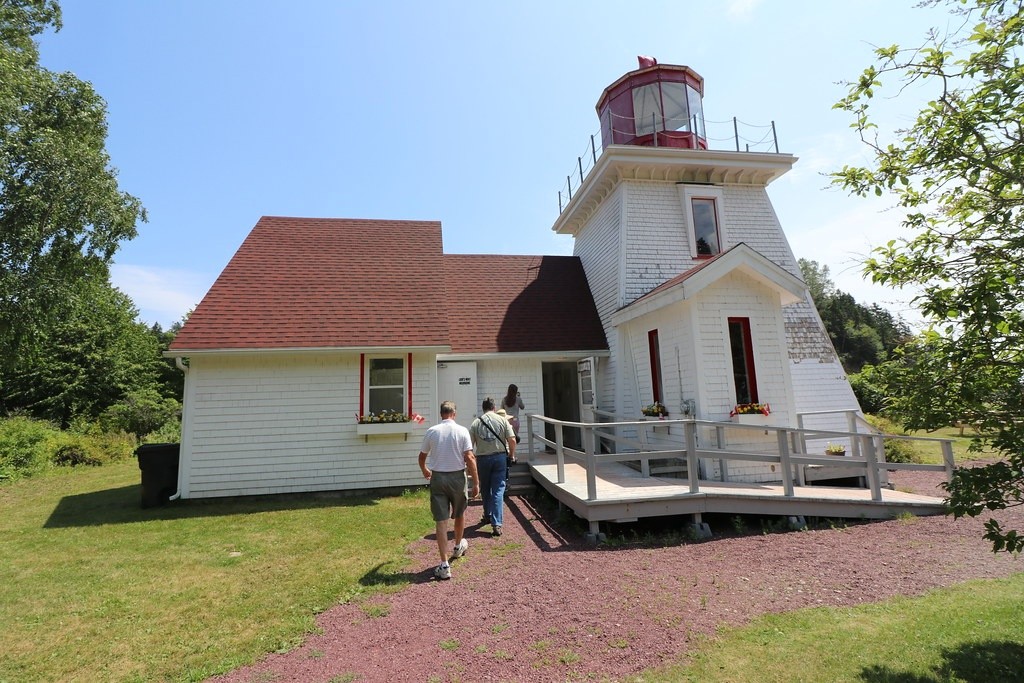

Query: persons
<box><xmin>418</xmin><ymin>400</ymin><xmax>479</xmax><ymax>580</ymax></box>
<box><xmin>469</xmin><ymin>398</ymin><xmax>515</xmax><ymax>534</ymax></box>
<box><xmin>501</xmin><ymin>384</ymin><xmax>525</xmax><ymax>462</ymax></box>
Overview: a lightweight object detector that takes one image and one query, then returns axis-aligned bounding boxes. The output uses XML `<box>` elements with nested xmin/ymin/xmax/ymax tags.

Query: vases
<box><xmin>357</xmin><ymin>423</ymin><xmax>413</xmax><ymax>433</ymax></box>
<box><xmin>731</xmin><ymin>414</ymin><xmax>771</xmax><ymax>425</ymax></box>
<box><xmin>644</xmin><ymin>411</ymin><xmax>669</xmax><ymax>416</ymax></box>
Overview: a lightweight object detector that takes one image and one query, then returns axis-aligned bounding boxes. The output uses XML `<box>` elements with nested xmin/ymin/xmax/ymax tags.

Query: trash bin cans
<box><xmin>132</xmin><ymin>442</ymin><xmax>180</xmax><ymax>501</ymax></box>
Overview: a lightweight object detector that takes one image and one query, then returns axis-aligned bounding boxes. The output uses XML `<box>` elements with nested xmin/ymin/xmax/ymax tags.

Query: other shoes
<box><xmin>506</xmin><ymin>455</ymin><xmax>518</xmax><ymax>467</ymax></box>
<box><xmin>482</xmin><ymin>513</ymin><xmax>492</xmax><ymax>523</ymax></box>
<box><xmin>493</xmin><ymin>525</ymin><xmax>503</xmax><ymax>536</ymax></box>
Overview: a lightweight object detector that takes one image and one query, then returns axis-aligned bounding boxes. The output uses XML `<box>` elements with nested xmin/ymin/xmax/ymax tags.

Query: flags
<box><xmin>412</xmin><ymin>414</ymin><xmax>425</xmax><ymax>424</ymax></box>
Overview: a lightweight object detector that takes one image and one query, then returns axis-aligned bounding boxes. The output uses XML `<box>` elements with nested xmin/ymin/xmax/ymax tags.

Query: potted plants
<box><xmin>824</xmin><ymin>442</ymin><xmax>847</xmax><ymax>456</ymax></box>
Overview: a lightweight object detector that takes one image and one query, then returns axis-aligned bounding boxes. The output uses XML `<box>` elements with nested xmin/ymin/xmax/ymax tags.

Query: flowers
<box><xmin>354</xmin><ymin>409</ymin><xmax>409</xmax><ymax>424</ymax></box>
<box><xmin>641</xmin><ymin>402</ymin><xmax>664</xmax><ymax>413</ymax></box>
<box><xmin>729</xmin><ymin>401</ymin><xmax>770</xmax><ymax>417</ymax></box>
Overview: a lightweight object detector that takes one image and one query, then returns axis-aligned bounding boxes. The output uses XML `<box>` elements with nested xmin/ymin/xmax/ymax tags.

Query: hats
<box><xmin>497</xmin><ymin>409</ymin><xmax>514</xmax><ymax>420</ymax></box>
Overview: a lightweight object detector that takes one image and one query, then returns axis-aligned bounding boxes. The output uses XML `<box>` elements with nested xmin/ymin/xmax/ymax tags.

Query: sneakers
<box><xmin>454</xmin><ymin>538</ymin><xmax>468</xmax><ymax>558</ymax></box>
<box><xmin>435</xmin><ymin>563</ymin><xmax>452</xmax><ymax>580</ymax></box>
